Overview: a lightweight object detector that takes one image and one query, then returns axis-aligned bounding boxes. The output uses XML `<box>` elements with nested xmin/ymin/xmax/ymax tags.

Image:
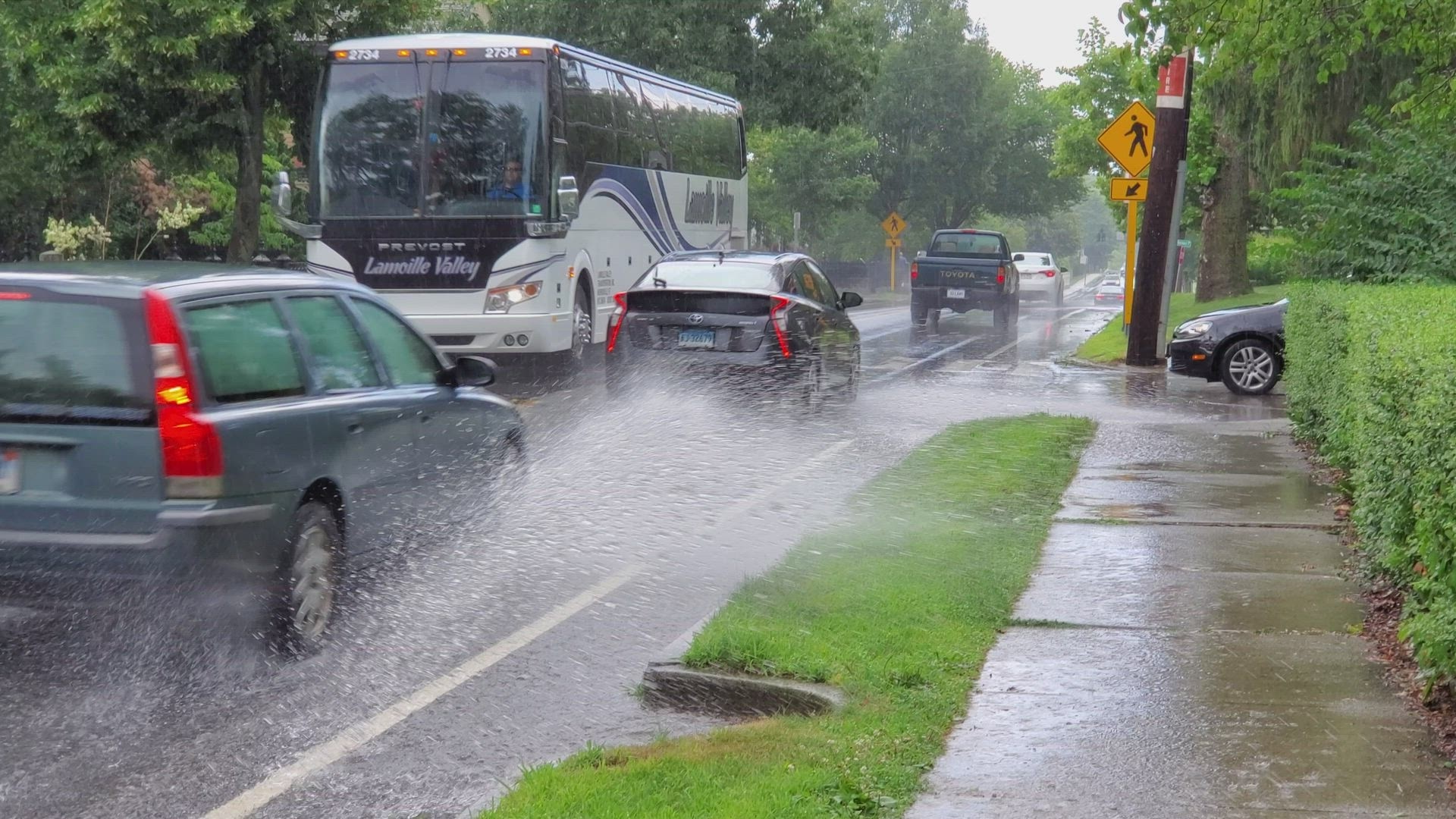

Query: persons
<box><xmin>486</xmin><ymin>157</ymin><xmax>534</xmax><ymax>199</ymax></box>
<box><xmin>642</xmin><ymin>150</ymin><xmax>667</xmax><ymax>170</ymax></box>
<box><xmin>899</xmin><ymin>252</ymin><xmax>909</xmax><ymax>288</ymax></box>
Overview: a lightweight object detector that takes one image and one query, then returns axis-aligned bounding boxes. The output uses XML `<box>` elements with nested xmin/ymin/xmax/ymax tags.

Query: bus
<box><xmin>269</xmin><ymin>31</ymin><xmax>748</xmax><ymax>389</ymax></box>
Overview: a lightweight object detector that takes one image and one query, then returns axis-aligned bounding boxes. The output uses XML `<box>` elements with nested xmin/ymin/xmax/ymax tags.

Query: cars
<box><xmin>605</xmin><ymin>248</ymin><xmax>865</xmax><ymax>418</ymax></box>
<box><xmin>1096</xmin><ymin>265</ymin><xmax>1135</xmax><ymax>305</ymax></box>
<box><xmin>1010</xmin><ymin>252</ymin><xmax>1068</xmax><ymax>304</ymax></box>
<box><xmin>1165</xmin><ymin>296</ymin><xmax>1290</xmax><ymax>397</ymax></box>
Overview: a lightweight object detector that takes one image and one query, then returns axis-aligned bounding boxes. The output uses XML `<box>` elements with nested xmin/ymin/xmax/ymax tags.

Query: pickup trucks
<box><xmin>910</xmin><ymin>229</ymin><xmax>1025</xmax><ymax>331</ymax></box>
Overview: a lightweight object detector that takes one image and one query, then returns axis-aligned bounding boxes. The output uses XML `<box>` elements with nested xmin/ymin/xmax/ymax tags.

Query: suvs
<box><xmin>0</xmin><ymin>262</ymin><xmax>531</xmax><ymax>666</ymax></box>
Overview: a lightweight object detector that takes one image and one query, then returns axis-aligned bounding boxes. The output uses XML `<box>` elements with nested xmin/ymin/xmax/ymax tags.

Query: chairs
<box><xmin>940</xmin><ymin>242</ymin><xmax>957</xmax><ymax>252</ymax></box>
<box><xmin>569</xmin><ymin>119</ymin><xmax>732</xmax><ymax>179</ymax></box>
<box><xmin>977</xmin><ymin>244</ymin><xmax>995</xmax><ymax>253</ymax></box>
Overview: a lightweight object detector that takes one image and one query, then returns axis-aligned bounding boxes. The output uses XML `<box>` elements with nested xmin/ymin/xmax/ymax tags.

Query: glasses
<box><xmin>504</xmin><ymin>166</ymin><xmax>522</xmax><ymax>172</ymax></box>
